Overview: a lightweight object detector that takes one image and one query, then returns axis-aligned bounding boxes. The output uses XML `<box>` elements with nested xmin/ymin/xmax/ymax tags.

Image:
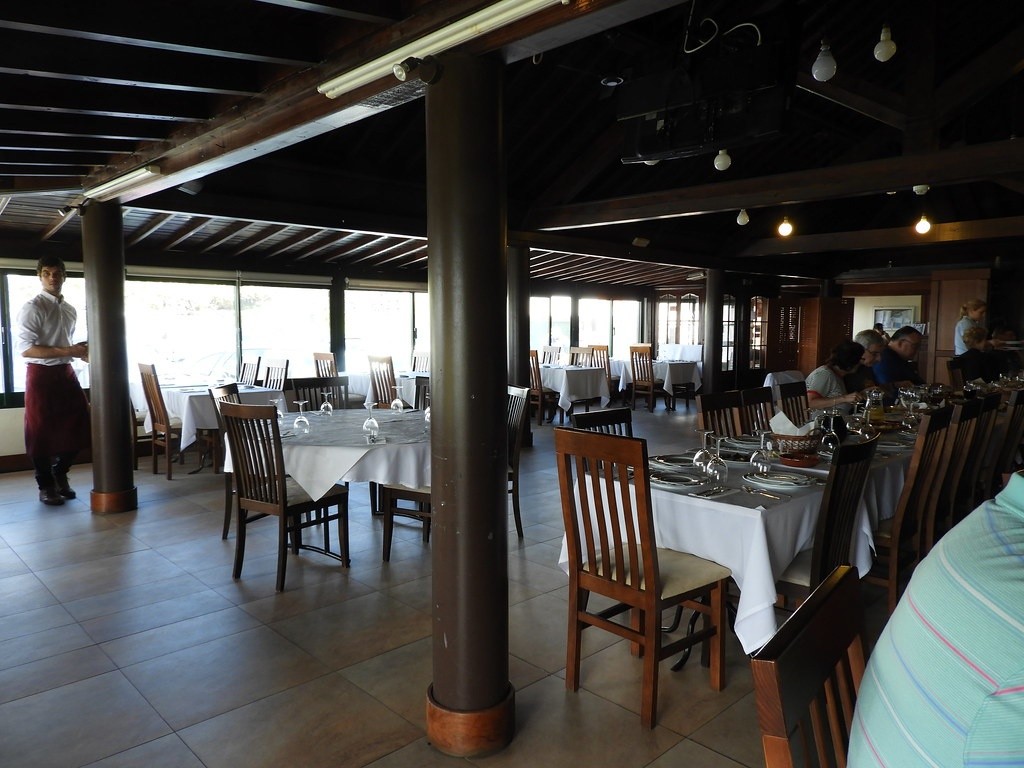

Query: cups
<box><xmin>991</xmin><ymin>329</ymin><xmax>1000</xmax><ymax>340</ymax></box>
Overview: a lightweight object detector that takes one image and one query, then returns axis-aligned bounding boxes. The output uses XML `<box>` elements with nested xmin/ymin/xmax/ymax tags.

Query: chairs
<box><xmin>131</xmin><ymin>344</ymin><xmax>1024</xmax><ymax>768</ymax></box>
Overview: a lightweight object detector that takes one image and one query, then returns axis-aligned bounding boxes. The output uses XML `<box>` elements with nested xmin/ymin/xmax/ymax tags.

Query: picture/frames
<box><xmin>873</xmin><ymin>306</ymin><xmax>914</xmax><ymax>332</ymax></box>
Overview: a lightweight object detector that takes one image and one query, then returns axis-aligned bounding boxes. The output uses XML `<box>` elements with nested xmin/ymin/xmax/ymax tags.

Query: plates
<box><xmin>645</xmin><ymin>411</ymin><xmax>915</xmax><ymax>490</ymax></box>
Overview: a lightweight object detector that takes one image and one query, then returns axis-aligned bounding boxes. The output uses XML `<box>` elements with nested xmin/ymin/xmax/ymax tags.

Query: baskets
<box><xmin>769</xmin><ymin>433</ymin><xmax>823</xmax><ymax>454</ymax></box>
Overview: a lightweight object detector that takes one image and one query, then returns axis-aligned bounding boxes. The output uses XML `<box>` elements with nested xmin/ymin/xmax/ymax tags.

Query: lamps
<box><xmin>316</xmin><ymin>0</ymin><xmax>566</xmax><ymax>100</ymax></box>
<box><xmin>57</xmin><ymin>165</ymin><xmax>161</xmax><ymax>216</ymax></box>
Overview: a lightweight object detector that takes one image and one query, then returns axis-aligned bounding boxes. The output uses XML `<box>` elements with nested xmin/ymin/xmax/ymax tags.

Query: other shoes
<box><xmin>51</xmin><ymin>463</ymin><xmax>75</xmax><ymax>499</ymax></box>
<box><xmin>40</xmin><ymin>487</ymin><xmax>64</xmax><ymax>505</ymax></box>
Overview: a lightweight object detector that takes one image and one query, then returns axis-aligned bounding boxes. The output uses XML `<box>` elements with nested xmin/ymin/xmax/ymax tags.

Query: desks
<box><xmin>223</xmin><ymin>409</ymin><xmax>431</xmax><ymax>564</ymax></box>
<box><xmin>721</xmin><ymin>347</ymin><xmax>734</xmax><ymax>371</ymax></box>
<box><xmin>530</xmin><ymin>363</ymin><xmax>611</xmax><ymax>424</ymax></box>
<box><xmin>560</xmin><ymin>417</ymin><xmax>919</xmax><ymax>672</ymax></box>
<box><xmin>338</xmin><ymin>368</ymin><xmax>429</xmax><ymax>410</ymax></box>
<box><xmin>610</xmin><ymin>357</ymin><xmax>701</xmax><ymax>412</ymax></box>
<box><xmin>130</xmin><ymin>384</ymin><xmax>287</xmax><ymax>473</ymax></box>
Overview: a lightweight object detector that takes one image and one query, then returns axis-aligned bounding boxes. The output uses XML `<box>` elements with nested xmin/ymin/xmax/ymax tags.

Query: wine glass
<box><xmin>320</xmin><ymin>392</ymin><xmax>334</xmax><ymax>416</ymax></box>
<box><xmin>291</xmin><ymin>400</ymin><xmax>310</xmax><ymax>436</ymax></box>
<box><xmin>423</xmin><ymin>392</ymin><xmax>432</xmax><ymax>431</ymax></box>
<box><xmin>390</xmin><ymin>386</ymin><xmax>404</xmax><ymax>412</ymax></box>
<box><xmin>693</xmin><ymin>372</ymin><xmax>1023</xmax><ymax>487</ymax></box>
<box><xmin>268</xmin><ymin>398</ymin><xmax>284</xmax><ymax>429</ymax></box>
<box><xmin>362</xmin><ymin>402</ymin><xmax>379</xmax><ymax>439</ymax></box>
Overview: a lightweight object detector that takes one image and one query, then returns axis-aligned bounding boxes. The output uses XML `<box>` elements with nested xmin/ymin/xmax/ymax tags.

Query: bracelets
<box><xmin>834</xmin><ymin>397</ymin><xmax>836</xmax><ymax>406</ymax></box>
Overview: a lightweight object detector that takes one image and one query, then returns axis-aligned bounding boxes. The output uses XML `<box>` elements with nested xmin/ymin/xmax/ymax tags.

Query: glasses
<box><xmin>859</xmin><ymin>358</ymin><xmax>865</xmax><ymax>363</ymax></box>
<box><xmin>867</xmin><ymin>349</ymin><xmax>880</xmax><ymax>357</ymax></box>
<box><xmin>903</xmin><ymin>339</ymin><xmax>921</xmax><ymax>349</ymax></box>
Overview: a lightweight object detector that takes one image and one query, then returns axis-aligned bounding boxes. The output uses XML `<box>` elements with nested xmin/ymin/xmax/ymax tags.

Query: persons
<box><xmin>950</xmin><ymin>327</ymin><xmax>1007</xmax><ymax>387</ymax></box>
<box><xmin>846</xmin><ymin>464</ymin><xmax>1024</xmax><ymax>768</ymax></box>
<box><xmin>18</xmin><ymin>254</ymin><xmax>88</xmax><ymax>504</ymax></box>
<box><xmin>804</xmin><ymin>341</ymin><xmax>881</xmax><ymax>418</ymax></box>
<box><xmin>883</xmin><ymin>326</ymin><xmax>927</xmax><ymax>390</ymax></box>
<box><xmin>953</xmin><ymin>299</ymin><xmax>987</xmax><ymax>358</ymax></box>
<box><xmin>992</xmin><ymin>327</ymin><xmax>1024</xmax><ymax>378</ymax></box>
<box><xmin>844</xmin><ymin>329</ymin><xmax>890</xmax><ymax>412</ymax></box>
<box><xmin>872</xmin><ymin>323</ymin><xmax>891</xmax><ymax>346</ymax></box>
